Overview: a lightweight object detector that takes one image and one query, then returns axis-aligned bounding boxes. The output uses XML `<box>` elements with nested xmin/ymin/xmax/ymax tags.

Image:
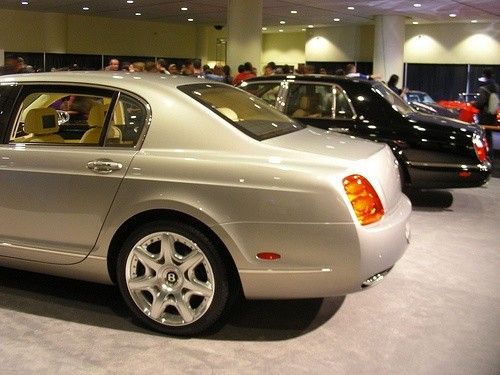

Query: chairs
<box><xmin>291</xmin><ymin>93</ymin><xmax>322</xmax><ymax>118</ymax></box>
<box><xmin>21</xmin><ymin>107</ymin><xmax>65</xmax><ymax>144</ymax></box>
<box><xmin>80</xmin><ymin>104</ymin><xmax>120</xmax><ymax>143</ymax></box>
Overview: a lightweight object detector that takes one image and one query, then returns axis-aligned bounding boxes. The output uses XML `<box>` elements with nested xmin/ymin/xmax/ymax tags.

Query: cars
<box><xmin>0</xmin><ymin>70</ymin><xmax>413</xmax><ymax>339</ymax></box>
<box><xmin>130</xmin><ymin>75</ymin><xmax>492</xmax><ymax>188</ymax></box>
<box><xmin>396</xmin><ymin>89</ymin><xmax>462</xmax><ymax>119</ymax></box>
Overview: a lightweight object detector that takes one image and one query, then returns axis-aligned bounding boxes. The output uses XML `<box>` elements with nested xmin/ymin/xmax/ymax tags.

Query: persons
<box><xmin>388</xmin><ymin>74</ymin><xmax>409</xmax><ymax>100</ymax></box>
<box><xmin>473</xmin><ymin>69</ymin><xmax>500</xmax><ymax>157</ymax></box>
<box><xmin>1</xmin><ymin>54</ymin><xmax>383</xmax><ymax>124</ymax></box>
